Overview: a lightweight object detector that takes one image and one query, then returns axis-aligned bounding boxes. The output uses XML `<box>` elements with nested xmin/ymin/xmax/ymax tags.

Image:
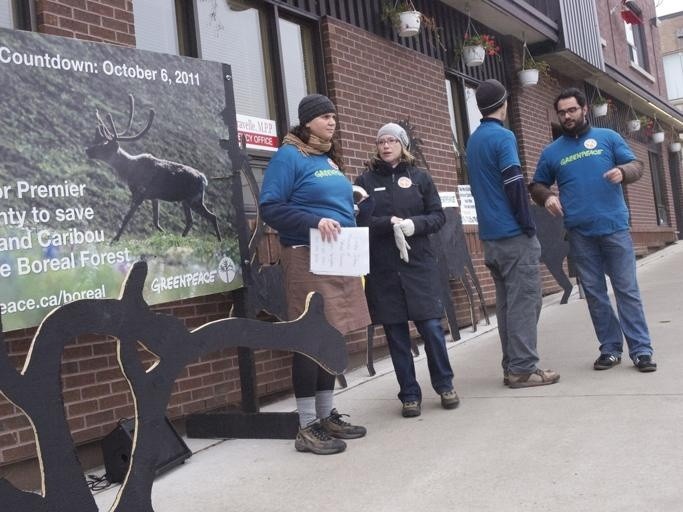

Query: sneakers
<box><xmin>321</xmin><ymin>408</ymin><xmax>366</xmax><ymax>439</ymax></box>
<box><xmin>441</xmin><ymin>388</ymin><xmax>459</xmax><ymax>408</ymax></box>
<box><xmin>633</xmin><ymin>352</ymin><xmax>657</xmax><ymax>372</ymax></box>
<box><xmin>594</xmin><ymin>352</ymin><xmax>621</xmax><ymax>370</ymax></box>
<box><xmin>295</xmin><ymin>418</ymin><xmax>347</xmax><ymax>454</ymax></box>
<box><xmin>402</xmin><ymin>399</ymin><xmax>421</xmax><ymax>416</ymax></box>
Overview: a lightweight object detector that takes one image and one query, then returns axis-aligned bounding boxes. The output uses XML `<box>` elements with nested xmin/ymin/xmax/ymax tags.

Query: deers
<box><xmin>85</xmin><ymin>93</ymin><xmax>224</xmax><ymax>247</ymax></box>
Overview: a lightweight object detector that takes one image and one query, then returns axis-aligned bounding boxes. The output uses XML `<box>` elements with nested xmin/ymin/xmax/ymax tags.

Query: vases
<box><xmin>461</xmin><ymin>46</ymin><xmax>485</xmax><ymax>68</ymax></box>
<box><xmin>625</xmin><ymin>119</ymin><xmax>641</xmax><ymax>132</ymax></box>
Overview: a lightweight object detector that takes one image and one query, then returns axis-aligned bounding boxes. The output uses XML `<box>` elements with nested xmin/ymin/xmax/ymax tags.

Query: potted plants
<box><xmin>508</xmin><ymin>31</ymin><xmax>561</xmax><ymax>92</ymax></box>
<box><xmin>665</xmin><ymin>122</ymin><xmax>683</xmax><ymax>153</ymax></box>
<box><xmin>586</xmin><ymin>76</ymin><xmax>618</xmax><ymax>121</ymax></box>
<box><xmin>378</xmin><ymin>0</ymin><xmax>448</xmax><ymax>57</ymax></box>
<box><xmin>644</xmin><ymin>110</ymin><xmax>665</xmax><ymax>144</ymax></box>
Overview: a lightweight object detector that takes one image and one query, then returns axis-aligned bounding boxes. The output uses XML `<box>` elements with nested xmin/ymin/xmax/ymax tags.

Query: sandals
<box><xmin>508</xmin><ymin>367</ymin><xmax>560</xmax><ymax>388</ymax></box>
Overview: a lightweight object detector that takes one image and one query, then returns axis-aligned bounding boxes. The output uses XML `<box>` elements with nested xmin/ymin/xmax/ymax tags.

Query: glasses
<box><xmin>557</xmin><ymin>107</ymin><xmax>581</xmax><ymax>116</ymax></box>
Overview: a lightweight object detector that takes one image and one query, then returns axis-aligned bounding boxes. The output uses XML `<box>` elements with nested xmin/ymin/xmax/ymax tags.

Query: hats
<box><xmin>475</xmin><ymin>78</ymin><xmax>507</xmax><ymax>115</ymax></box>
<box><xmin>376</xmin><ymin>122</ymin><xmax>409</xmax><ymax>147</ymax></box>
<box><xmin>298</xmin><ymin>94</ymin><xmax>337</xmax><ymax>126</ymax></box>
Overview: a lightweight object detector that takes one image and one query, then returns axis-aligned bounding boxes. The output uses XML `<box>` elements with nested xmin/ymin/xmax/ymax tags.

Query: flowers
<box><xmin>641</xmin><ymin>115</ymin><xmax>654</xmax><ymax>130</ymax></box>
<box><xmin>452</xmin><ymin>33</ymin><xmax>501</xmax><ymax>61</ymax></box>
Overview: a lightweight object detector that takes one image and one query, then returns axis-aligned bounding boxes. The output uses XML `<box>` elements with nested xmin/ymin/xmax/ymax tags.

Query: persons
<box><xmin>465</xmin><ymin>78</ymin><xmax>561</xmax><ymax>389</ymax></box>
<box><xmin>527</xmin><ymin>89</ymin><xmax>658</xmax><ymax>373</ymax></box>
<box><xmin>353</xmin><ymin>121</ymin><xmax>460</xmax><ymax>418</ymax></box>
<box><xmin>257</xmin><ymin>94</ymin><xmax>368</xmax><ymax>456</ymax></box>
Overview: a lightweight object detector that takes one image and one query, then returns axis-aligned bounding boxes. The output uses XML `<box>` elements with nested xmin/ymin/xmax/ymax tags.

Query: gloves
<box><xmin>392</xmin><ymin>224</ymin><xmax>411</xmax><ymax>264</ymax></box>
<box><xmin>400</xmin><ymin>218</ymin><xmax>415</xmax><ymax>237</ymax></box>
